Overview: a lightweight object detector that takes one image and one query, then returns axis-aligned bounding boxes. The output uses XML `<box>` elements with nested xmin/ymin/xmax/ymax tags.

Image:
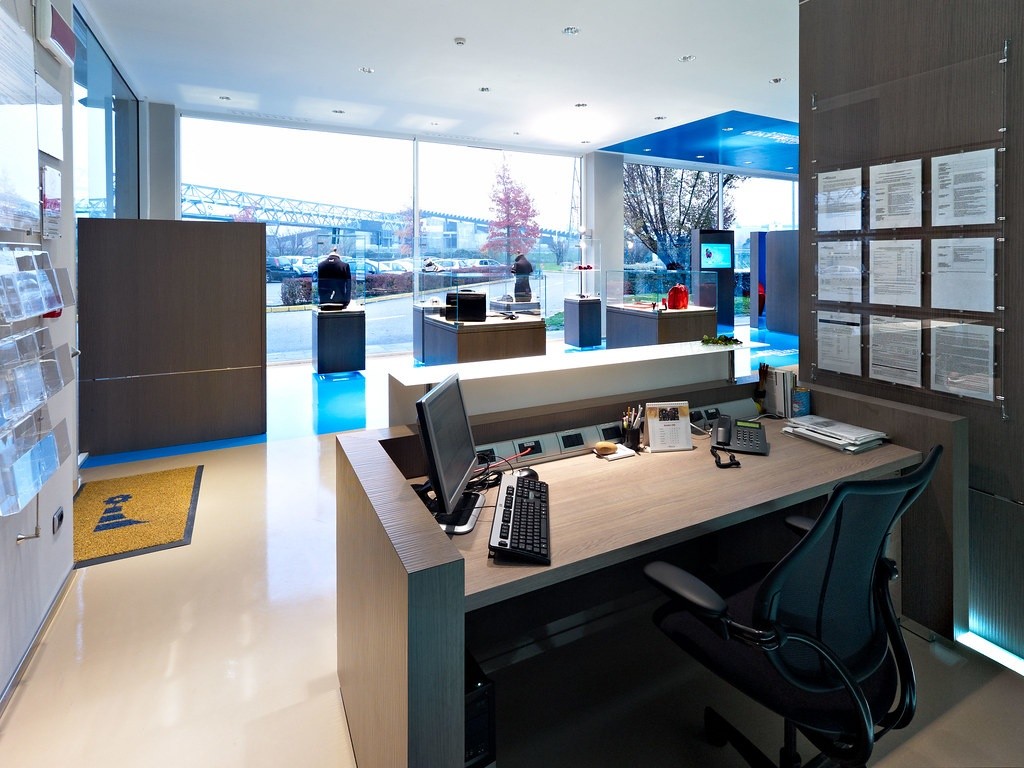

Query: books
<box><xmin>766</xmin><ymin>368</ymin><xmax>797</xmax><ymax>418</ymax></box>
<box><xmin>781</xmin><ymin>415</ymin><xmax>888</xmax><ymax>454</ymax></box>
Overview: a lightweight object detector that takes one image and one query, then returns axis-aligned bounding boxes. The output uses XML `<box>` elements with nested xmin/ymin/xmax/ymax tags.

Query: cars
<box><xmin>316</xmin><ymin>254</ymin><xmax>442</xmax><ymax>281</ymax></box>
<box><xmin>469</xmin><ymin>258</ymin><xmax>508</xmax><ymax>273</ymax></box>
<box><xmin>435</xmin><ymin>260</ymin><xmax>474</xmax><ymax>273</ymax></box>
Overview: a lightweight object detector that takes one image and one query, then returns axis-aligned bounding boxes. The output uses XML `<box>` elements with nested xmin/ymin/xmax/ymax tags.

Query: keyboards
<box><xmin>488</xmin><ymin>474</ymin><xmax>551</xmax><ymax>566</ymax></box>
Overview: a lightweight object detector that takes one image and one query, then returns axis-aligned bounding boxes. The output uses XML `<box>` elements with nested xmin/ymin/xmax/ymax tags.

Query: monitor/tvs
<box><xmin>416</xmin><ymin>373</ymin><xmax>485</xmax><ymax>533</ymax></box>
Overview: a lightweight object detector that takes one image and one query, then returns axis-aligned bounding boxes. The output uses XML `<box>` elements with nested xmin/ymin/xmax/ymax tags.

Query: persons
<box><xmin>511</xmin><ymin>253</ymin><xmax>532</xmax><ymax>301</ymax></box>
<box><xmin>706</xmin><ymin>249</ymin><xmax>712</xmax><ymax>258</ymax></box>
<box><xmin>318</xmin><ymin>251</ymin><xmax>352</xmax><ymax>311</ymax></box>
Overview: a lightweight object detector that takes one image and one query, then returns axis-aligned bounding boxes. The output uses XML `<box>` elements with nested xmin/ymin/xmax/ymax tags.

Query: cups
<box><xmin>792</xmin><ymin>388</ymin><xmax>811</xmax><ymax>417</ymax></box>
<box><xmin>622</xmin><ymin>428</ymin><xmax>640</xmax><ymax>449</ymax></box>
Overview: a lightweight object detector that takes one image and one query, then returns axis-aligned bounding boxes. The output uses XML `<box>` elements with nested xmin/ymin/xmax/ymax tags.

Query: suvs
<box><xmin>279</xmin><ymin>256</ymin><xmax>318</xmax><ymax>279</ymax></box>
<box><xmin>266</xmin><ymin>256</ymin><xmax>295</xmax><ymax>283</ymax></box>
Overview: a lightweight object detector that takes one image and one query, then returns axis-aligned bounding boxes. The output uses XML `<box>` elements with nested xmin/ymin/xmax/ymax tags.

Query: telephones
<box><xmin>710</xmin><ymin>414</ymin><xmax>769</xmax><ymax>455</ymax></box>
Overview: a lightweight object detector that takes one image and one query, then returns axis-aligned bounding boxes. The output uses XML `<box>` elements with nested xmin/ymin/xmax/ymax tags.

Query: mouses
<box><xmin>510</xmin><ymin>468</ymin><xmax>538</xmax><ymax>480</ymax></box>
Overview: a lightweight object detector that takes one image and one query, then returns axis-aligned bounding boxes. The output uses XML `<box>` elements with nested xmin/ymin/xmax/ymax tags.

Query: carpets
<box><xmin>73</xmin><ymin>465</ymin><xmax>204</xmax><ymax>569</ymax></box>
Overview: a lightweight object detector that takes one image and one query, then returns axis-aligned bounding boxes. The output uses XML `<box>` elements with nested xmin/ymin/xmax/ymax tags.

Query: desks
<box><xmin>336</xmin><ymin>341</ymin><xmax>969</xmax><ymax>768</ymax></box>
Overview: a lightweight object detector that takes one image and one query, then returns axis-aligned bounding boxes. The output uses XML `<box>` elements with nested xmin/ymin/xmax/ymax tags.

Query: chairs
<box><xmin>640</xmin><ymin>444</ymin><xmax>942</xmax><ymax>768</ymax></box>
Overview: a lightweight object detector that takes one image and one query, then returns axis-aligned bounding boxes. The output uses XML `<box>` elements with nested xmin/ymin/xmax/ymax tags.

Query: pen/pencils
<box><xmin>622</xmin><ymin>403</ymin><xmax>644</xmax><ymax>430</ymax></box>
